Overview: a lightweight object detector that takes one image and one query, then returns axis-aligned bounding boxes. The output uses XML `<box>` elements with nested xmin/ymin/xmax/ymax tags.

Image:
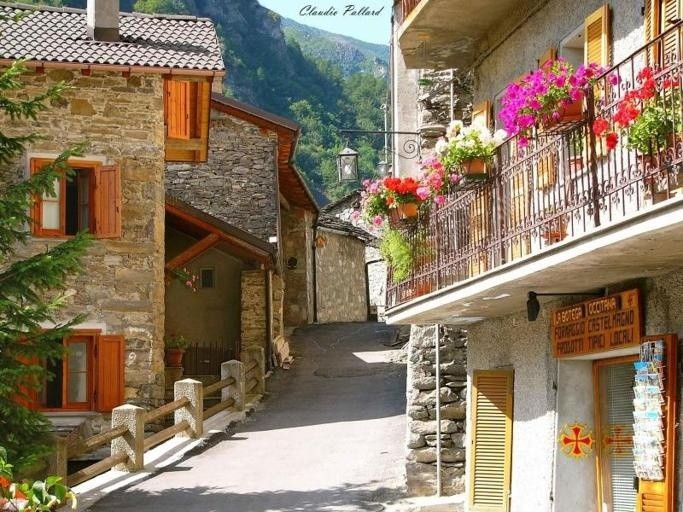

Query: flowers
<box><xmin>352</xmin><ymin>117</ymin><xmax>509</xmax><ymax>227</ymax></box>
<box><xmin>165</xmin><ymin>265</ymin><xmax>201</xmax><ymax>294</ymax></box>
<box><xmin>496</xmin><ymin>53</ymin><xmax>683</xmax><ymax>155</ymax></box>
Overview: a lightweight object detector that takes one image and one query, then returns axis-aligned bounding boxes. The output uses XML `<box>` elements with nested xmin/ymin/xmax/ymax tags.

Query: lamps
<box><xmin>335</xmin><ymin>128</ymin><xmax>418</xmax><ymax>183</ymax></box>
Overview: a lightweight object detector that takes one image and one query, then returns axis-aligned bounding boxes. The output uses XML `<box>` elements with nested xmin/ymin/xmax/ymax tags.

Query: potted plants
<box><xmin>164</xmin><ymin>329</ymin><xmax>193</xmax><ymax>365</ymax></box>
